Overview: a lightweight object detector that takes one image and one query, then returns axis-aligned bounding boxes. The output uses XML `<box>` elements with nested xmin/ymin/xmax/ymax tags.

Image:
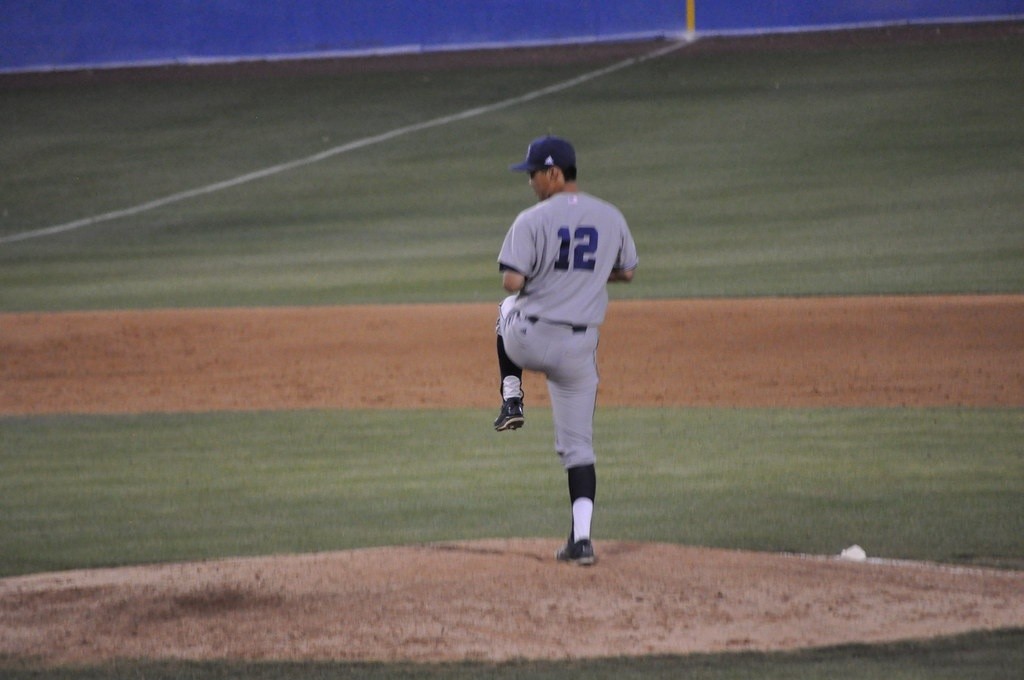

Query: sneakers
<box><xmin>557</xmin><ymin>538</ymin><xmax>594</xmax><ymax>565</ymax></box>
<box><xmin>494</xmin><ymin>396</ymin><xmax>524</xmax><ymax>431</ymax></box>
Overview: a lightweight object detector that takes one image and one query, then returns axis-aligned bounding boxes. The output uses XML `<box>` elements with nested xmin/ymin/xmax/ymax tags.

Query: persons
<box><xmin>494</xmin><ymin>135</ymin><xmax>641</xmax><ymax>566</ymax></box>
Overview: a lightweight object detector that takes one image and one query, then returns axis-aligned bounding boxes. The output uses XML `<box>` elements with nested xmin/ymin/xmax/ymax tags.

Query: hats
<box><xmin>513</xmin><ymin>137</ymin><xmax>576</xmax><ymax>171</ymax></box>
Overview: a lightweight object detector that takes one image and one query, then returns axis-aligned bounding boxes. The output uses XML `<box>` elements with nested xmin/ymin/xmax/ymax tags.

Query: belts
<box><xmin>527</xmin><ymin>316</ymin><xmax>587</xmax><ymax>333</ymax></box>
